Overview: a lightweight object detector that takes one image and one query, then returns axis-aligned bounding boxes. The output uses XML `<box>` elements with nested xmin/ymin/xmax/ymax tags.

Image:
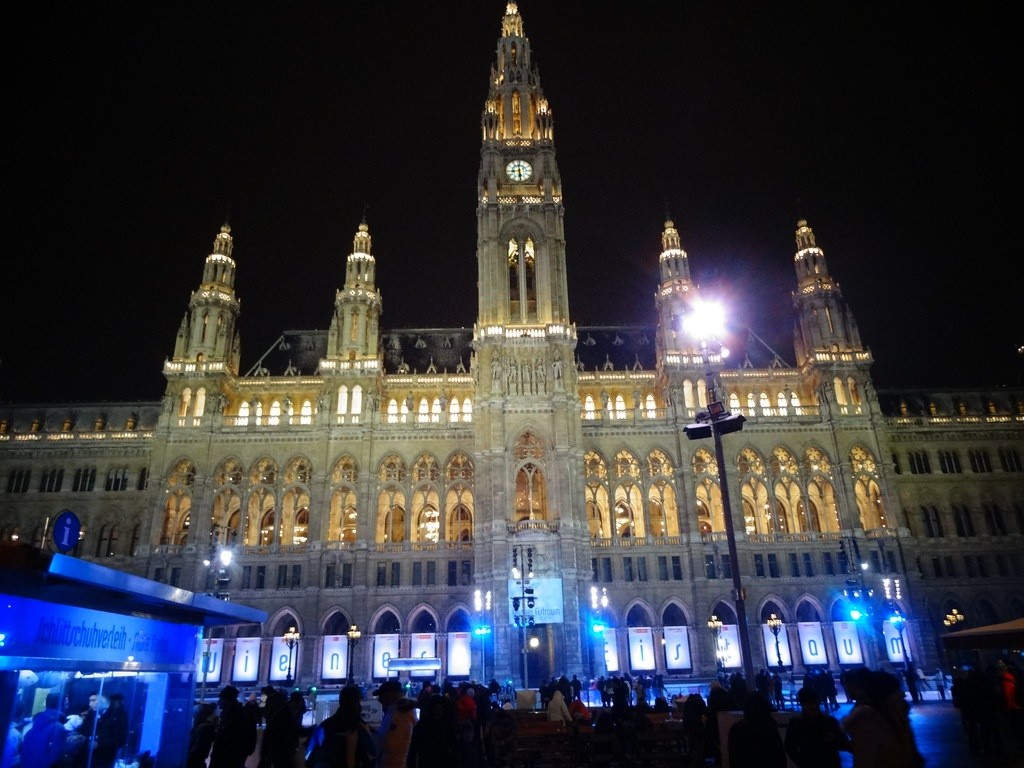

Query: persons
<box><xmin>15</xmin><ymin>647</ymin><xmax>1024</xmax><ymax>765</ymax></box>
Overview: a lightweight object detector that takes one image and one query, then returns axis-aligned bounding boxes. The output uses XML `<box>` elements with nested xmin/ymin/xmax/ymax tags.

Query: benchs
<box><xmin>505</xmin><ymin>708</ymin><xmax>690</xmax><ymax>768</ymax></box>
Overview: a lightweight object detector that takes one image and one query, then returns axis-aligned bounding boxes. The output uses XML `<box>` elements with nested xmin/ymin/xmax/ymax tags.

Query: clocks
<box><xmin>504</xmin><ymin>159</ymin><xmax>535</xmax><ymax>185</ymax></box>
